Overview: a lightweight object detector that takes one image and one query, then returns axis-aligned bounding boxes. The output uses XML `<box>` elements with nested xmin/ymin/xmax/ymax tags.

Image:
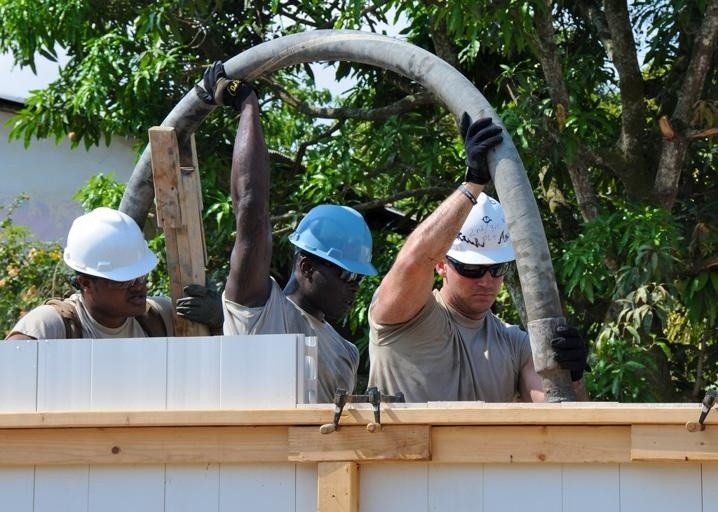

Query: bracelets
<box><xmin>459</xmin><ymin>185</ymin><xmax>478</xmax><ymax>205</ymax></box>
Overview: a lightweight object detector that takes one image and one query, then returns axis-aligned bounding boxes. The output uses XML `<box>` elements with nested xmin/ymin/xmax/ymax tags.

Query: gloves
<box><xmin>176</xmin><ymin>284</ymin><xmax>224</xmax><ymax>330</ymax></box>
<box><xmin>194</xmin><ymin>59</ymin><xmax>253</xmax><ymax>111</ymax></box>
<box><xmin>549</xmin><ymin>324</ymin><xmax>591</xmax><ymax>382</ymax></box>
<box><xmin>458</xmin><ymin>111</ymin><xmax>503</xmax><ymax>186</ymax></box>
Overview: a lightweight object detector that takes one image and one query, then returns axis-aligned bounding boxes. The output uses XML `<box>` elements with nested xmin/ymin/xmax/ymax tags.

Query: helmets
<box><xmin>287</xmin><ymin>203</ymin><xmax>379</xmax><ymax>278</ymax></box>
<box><xmin>62</xmin><ymin>207</ymin><xmax>161</xmax><ymax>282</ymax></box>
<box><xmin>445</xmin><ymin>192</ymin><xmax>516</xmax><ymax>266</ymax></box>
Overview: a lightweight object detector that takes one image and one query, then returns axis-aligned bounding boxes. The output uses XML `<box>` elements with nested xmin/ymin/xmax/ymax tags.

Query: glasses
<box><xmin>445</xmin><ymin>255</ymin><xmax>510</xmax><ymax>279</ymax></box>
<box><xmin>107</xmin><ymin>274</ymin><xmax>149</xmax><ymax>291</ymax></box>
<box><xmin>312</xmin><ymin>253</ymin><xmax>366</xmax><ymax>284</ymax></box>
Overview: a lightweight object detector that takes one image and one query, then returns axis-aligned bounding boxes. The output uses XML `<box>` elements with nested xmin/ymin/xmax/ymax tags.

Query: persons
<box><xmin>196</xmin><ymin>57</ymin><xmax>380</xmax><ymax>403</ymax></box>
<box><xmin>5</xmin><ymin>204</ymin><xmax>223</xmax><ymax>338</ymax></box>
<box><xmin>365</xmin><ymin>112</ymin><xmax>591</xmax><ymax>401</ymax></box>
